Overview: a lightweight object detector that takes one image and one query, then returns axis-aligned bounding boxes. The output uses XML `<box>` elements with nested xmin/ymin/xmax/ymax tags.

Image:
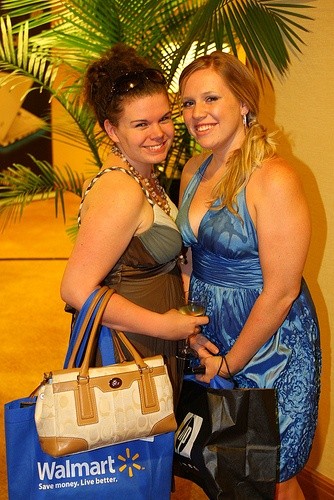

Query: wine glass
<box><xmin>176</xmin><ymin>290</ymin><xmax>205</xmax><ymax>360</ymax></box>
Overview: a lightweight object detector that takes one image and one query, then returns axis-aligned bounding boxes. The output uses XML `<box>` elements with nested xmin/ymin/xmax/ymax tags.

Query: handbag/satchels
<box><xmin>35</xmin><ymin>288</ymin><xmax>177</xmax><ymax>457</ymax></box>
<box><xmin>175</xmin><ymin>356</ymin><xmax>279</xmax><ymax>500</ymax></box>
<box><xmin>4</xmin><ymin>286</ymin><xmax>177</xmax><ymax>500</ymax></box>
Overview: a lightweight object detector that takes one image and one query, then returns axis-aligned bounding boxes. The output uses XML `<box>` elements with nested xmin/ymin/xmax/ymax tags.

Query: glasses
<box><xmin>106</xmin><ymin>68</ymin><xmax>166</xmax><ymax>106</ymax></box>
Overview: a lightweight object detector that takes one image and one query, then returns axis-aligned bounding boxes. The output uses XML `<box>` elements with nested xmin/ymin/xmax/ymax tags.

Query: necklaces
<box><xmin>111</xmin><ymin>145</ymin><xmax>171</xmax><ymax>216</ymax></box>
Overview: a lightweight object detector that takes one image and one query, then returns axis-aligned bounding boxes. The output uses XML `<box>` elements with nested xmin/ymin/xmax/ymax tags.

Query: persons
<box><xmin>60</xmin><ymin>44</ymin><xmax>209</xmax><ymax>414</ymax></box>
<box><xmin>174</xmin><ymin>50</ymin><xmax>321</xmax><ymax>500</ymax></box>
